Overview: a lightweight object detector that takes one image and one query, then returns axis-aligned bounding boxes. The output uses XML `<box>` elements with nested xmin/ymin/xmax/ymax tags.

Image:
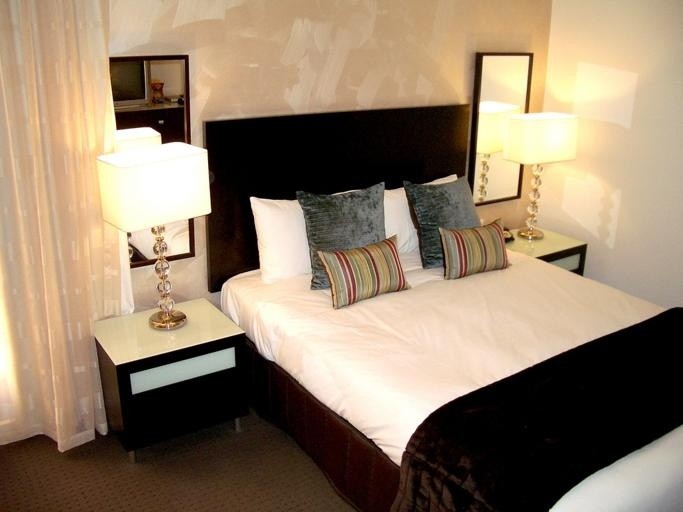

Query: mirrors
<box><xmin>104</xmin><ymin>54</ymin><xmax>195</xmax><ymax>267</ymax></box>
<box><xmin>468</xmin><ymin>48</ymin><xmax>535</xmax><ymax>207</ymax></box>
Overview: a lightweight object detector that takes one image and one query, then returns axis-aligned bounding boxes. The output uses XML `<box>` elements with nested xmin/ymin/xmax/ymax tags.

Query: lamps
<box><xmin>477</xmin><ymin>97</ymin><xmax>520</xmax><ymax>200</ymax></box>
<box><xmin>511</xmin><ymin>112</ymin><xmax>578</xmax><ymax>239</ymax></box>
<box><xmin>102</xmin><ymin>137</ymin><xmax>210</xmax><ymax>331</ymax></box>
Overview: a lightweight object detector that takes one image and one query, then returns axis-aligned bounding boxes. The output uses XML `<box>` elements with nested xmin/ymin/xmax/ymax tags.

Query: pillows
<box><xmin>435</xmin><ymin>216</ymin><xmax>518</xmax><ymax>280</ymax></box>
<box><xmin>405</xmin><ymin>175</ymin><xmax>485</xmax><ymax>272</ymax></box>
<box><xmin>248</xmin><ymin>184</ymin><xmax>384</xmax><ymax>283</ymax></box>
<box><xmin>312</xmin><ymin>236</ymin><xmax>410</xmax><ymax>312</ymax></box>
<box><xmin>294</xmin><ymin>182</ymin><xmax>389</xmax><ymax>294</ymax></box>
<box><xmin>384</xmin><ymin>175</ymin><xmax>461</xmax><ymax>270</ymax></box>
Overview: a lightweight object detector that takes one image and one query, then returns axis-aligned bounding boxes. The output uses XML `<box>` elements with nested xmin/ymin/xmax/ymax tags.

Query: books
<box><xmin>164</xmin><ymin>94</ymin><xmax>183</xmax><ymax>102</ymax></box>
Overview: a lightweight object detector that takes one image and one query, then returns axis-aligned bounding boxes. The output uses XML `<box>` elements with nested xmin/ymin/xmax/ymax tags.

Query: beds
<box><xmin>201</xmin><ymin>101</ymin><xmax>683</xmax><ymax>511</ymax></box>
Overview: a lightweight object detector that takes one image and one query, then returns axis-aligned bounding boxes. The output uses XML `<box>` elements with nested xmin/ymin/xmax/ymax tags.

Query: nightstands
<box><xmin>507</xmin><ymin>226</ymin><xmax>586</xmax><ymax>280</ymax></box>
<box><xmin>94</xmin><ymin>291</ymin><xmax>247</xmax><ymax>462</ymax></box>
